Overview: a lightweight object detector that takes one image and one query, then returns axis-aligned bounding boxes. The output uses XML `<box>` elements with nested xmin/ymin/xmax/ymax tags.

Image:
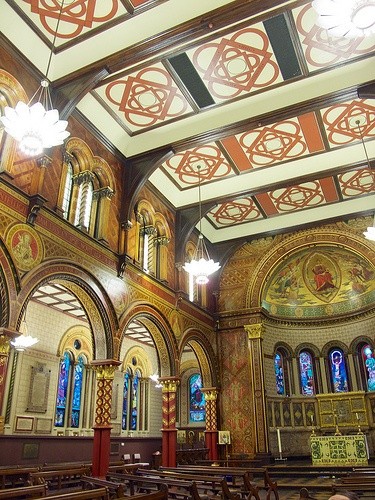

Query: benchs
<box><xmin>0</xmin><ymin>462</ymin><xmax>375</xmax><ymax>500</ymax></box>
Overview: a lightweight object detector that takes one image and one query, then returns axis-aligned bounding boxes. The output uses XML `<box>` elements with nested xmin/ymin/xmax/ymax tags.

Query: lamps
<box><xmin>1</xmin><ymin>0</ymin><xmax>70</xmax><ymax>151</ymax></box>
<box><xmin>355</xmin><ymin>120</ymin><xmax>375</xmax><ymax>241</ymax></box>
<box><xmin>182</xmin><ymin>164</ymin><xmax>221</xmax><ymax>285</ymax></box>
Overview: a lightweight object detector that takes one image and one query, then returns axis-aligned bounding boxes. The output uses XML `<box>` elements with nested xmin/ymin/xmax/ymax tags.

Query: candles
<box><xmin>277</xmin><ymin>429</ymin><xmax>282</xmax><ymax>453</ymax></box>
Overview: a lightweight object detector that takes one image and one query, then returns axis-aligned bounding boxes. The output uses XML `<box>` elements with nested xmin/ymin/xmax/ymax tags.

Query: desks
<box><xmin>310</xmin><ymin>435</ymin><xmax>369</xmax><ymax>466</ymax></box>
<box><xmin>201</xmin><ymin>459</ymin><xmax>262</xmax><ymax>481</ymax></box>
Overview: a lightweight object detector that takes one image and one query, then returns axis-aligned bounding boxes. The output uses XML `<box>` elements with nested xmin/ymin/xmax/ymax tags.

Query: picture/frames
<box><xmin>23</xmin><ymin>443</ymin><xmax>39</xmax><ymax>460</ymax></box>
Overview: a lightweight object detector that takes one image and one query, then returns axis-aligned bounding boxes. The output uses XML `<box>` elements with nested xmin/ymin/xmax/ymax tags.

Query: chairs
<box><xmin>123</xmin><ymin>453</ymin><xmax>150</xmax><ymax>472</ymax></box>
